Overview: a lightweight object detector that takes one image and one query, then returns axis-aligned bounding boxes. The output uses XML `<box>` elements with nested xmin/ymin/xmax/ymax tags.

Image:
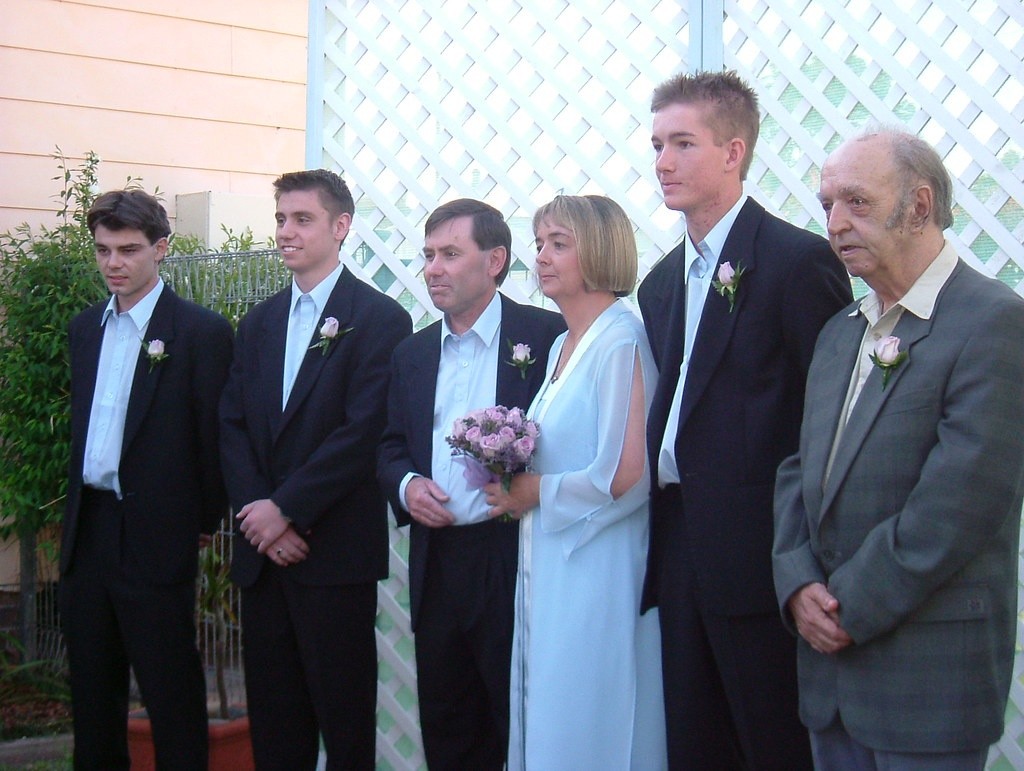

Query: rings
<box><xmin>276</xmin><ymin>548</ymin><xmax>282</xmax><ymax>554</ymax></box>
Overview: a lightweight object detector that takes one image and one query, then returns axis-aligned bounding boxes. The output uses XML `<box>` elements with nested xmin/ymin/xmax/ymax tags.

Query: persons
<box><xmin>214</xmin><ymin>169</ymin><xmax>413</xmax><ymax>771</ymax></box>
<box><xmin>375</xmin><ymin>200</ymin><xmax>568</xmax><ymax>771</ymax></box>
<box><xmin>635</xmin><ymin>73</ymin><xmax>854</xmax><ymax>771</ymax></box>
<box><xmin>63</xmin><ymin>192</ymin><xmax>235</xmax><ymax>771</ymax></box>
<box><xmin>482</xmin><ymin>196</ymin><xmax>666</xmax><ymax>771</ymax></box>
<box><xmin>774</xmin><ymin>131</ymin><xmax>1024</xmax><ymax>771</ymax></box>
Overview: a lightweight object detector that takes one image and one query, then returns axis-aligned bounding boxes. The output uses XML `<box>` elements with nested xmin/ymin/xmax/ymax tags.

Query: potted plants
<box><xmin>129</xmin><ymin>545</ymin><xmax>253</xmax><ymax>771</ymax></box>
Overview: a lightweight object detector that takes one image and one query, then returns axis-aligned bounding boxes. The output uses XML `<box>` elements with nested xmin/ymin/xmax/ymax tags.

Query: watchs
<box><xmin>278</xmin><ymin>506</ymin><xmax>293</xmax><ymax>523</ymax></box>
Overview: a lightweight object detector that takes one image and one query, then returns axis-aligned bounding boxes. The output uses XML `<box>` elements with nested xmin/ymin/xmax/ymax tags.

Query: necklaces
<box><xmin>550</xmin><ymin>309</ymin><xmax>607</xmax><ymax>384</ymax></box>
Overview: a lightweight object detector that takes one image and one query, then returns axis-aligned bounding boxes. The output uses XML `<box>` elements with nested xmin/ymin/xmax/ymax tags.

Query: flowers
<box><xmin>504</xmin><ymin>338</ymin><xmax>536</xmax><ymax>379</ymax></box>
<box><xmin>308</xmin><ymin>316</ymin><xmax>354</xmax><ymax>357</ymax></box>
<box><xmin>867</xmin><ymin>336</ymin><xmax>909</xmax><ymax>392</ymax></box>
<box><xmin>443</xmin><ymin>404</ymin><xmax>542</xmax><ymax>524</ymax></box>
<box><xmin>710</xmin><ymin>260</ymin><xmax>748</xmax><ymax>314</ymax></box>
<box><xmin>137</xmin><ymin>335</ymin><xmax>170</xmax><ymax>374</ymax></box>
